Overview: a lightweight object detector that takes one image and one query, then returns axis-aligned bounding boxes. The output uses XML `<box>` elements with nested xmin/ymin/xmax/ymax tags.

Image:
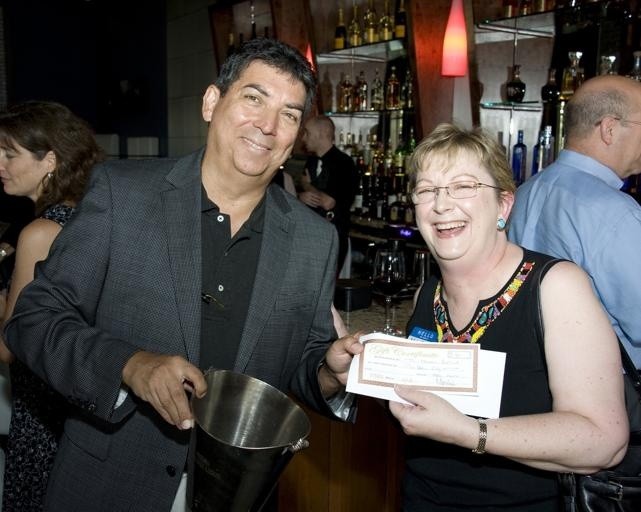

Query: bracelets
<box><xmin>301</xmin><ymin>181</ymin><xmax>311</xmax><ymax>184</ymax></box>
<box><xmin>472</xmin><ymin>418</ymin><xmax>491</xmax><ymax>456</ymax></box>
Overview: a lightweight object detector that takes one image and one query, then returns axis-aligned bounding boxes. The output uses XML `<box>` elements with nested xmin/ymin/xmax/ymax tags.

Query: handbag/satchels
<box><xmin>530</xmin><ymin>258</ymin><xmax>640</xmax><ymax>511</ymax></box>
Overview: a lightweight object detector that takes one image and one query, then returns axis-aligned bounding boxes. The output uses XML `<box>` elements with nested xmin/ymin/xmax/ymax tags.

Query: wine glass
<box><xmin>371</xmin><ymin>249</ymin><xmax>407</xmax><ymax>336</ymax></box>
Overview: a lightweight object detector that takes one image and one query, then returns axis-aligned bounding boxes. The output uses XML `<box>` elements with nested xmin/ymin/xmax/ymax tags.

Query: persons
<box><xmin>297</xmin><ymin>114</ymin><xmax>359</xmax><ymax>287</ymax></box>
<box><xmin>1</xmin><ymin>101</ymin><xmax>106</xmax><ymax>511</ymax></box>
<box><xmin>332</xmin><ymin>120</ymin><xmax>630</xmax><ymax>512</ymax></box>
<box><xmin>1</xmin><ymin>37</ymin><xmax>367</xmax><ymax>511</ymax></box>
<box><xmin>505</xmin><ymin>75</ymin><xmax>641</xmax><ymax>511</ymax></box>
<box><xmin>267</xmin><ymin>168</ymin><xmax>300</xmax><ymax>200</ymax></box>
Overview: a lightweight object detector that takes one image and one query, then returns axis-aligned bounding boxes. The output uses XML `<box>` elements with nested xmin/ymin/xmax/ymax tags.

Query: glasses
<box><xmin>409</xmin><ymin>179</ymin><xmax>500</xmax><ymax>204</ymax></box>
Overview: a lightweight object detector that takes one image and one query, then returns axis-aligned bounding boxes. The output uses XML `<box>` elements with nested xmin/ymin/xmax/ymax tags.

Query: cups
<box><xmin>412</xmin><ymin>250</ymin><xmax>432</xmax><ymax>286</ymax></box>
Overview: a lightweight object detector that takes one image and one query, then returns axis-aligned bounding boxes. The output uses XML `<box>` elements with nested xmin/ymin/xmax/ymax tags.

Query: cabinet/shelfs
<box><xmin>457</xmin><ymin>1</ymin><xmax>640</xmax><ymax>202</ymax></box>
<box><xmin>206</xmin><ymin>1</ymin><xmax>322</xmax><ymax>164</ymax></box>
<box><xmin>300</xmin><ymin>0</ymin><xmax>460</xmax><ymax>238</ymax></box>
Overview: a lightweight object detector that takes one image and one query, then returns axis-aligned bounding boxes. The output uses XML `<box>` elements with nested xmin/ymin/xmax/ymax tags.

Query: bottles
<box><xmin>629</xmin><ymin>185</ymin><xmax>641</xmax><ymax>202</ymax></box>
<box><xmin>496</xmin><ymin>131</ymin><xmax>507</xmax><ymax>155</ymax></box>
<box><xmin>319</xmin><ymin>72</ymin><xmax>333</xmax><ymax>113</ymax></box>
<box><xmin>502</xmin><ymin>0</ymin><xmax>548</xmax><ymax>20</ymax></box>
<box><xmin>538</xmin><ymin>49</ymin><xmax>641</xmax><ymax>103</ymax></box>
<box><xmin>334</xmin><ymin>0</ymin><xmax>407</xmax><ymax>51</ymax></box>
<box><xmin>336</xmin><ymin>126</ymin><xmax>416</xmax><ymax>226</ymax></box>
<box><xmin>336</xmin><ymin>66</ymin><xmax>413</xmax><ymax>113</ymax></box>
<box><xmin>505</xmin><ymin>62</ymin><xmax>527</xmax><ymax>103</ymax></box>
<box><xmin>532</xmin><ymin>126</ymin><xmax>555</xmax><ymax>176</ymax></box>
<box><xmin>511</xmin><ymin>130</ymin><xmax>529</xmax><ymax>186</ymax></box>
<box><xmin>226</xmin><ymin>22</ymin><xmax>271</xmax><ymax>58</ymax></box>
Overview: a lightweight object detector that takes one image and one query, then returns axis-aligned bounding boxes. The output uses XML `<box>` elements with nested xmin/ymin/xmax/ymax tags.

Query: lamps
<box><xmin>439</xmin><ymin>1</ymin><xmax>471</xmax><ymax>80</ymax></box>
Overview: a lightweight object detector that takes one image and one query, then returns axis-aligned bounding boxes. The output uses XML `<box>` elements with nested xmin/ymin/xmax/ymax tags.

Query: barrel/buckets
<box><xmin>184</xmin><ymin>368</ymin><xmax>313</xmax><ymax>512</ymax></box>
